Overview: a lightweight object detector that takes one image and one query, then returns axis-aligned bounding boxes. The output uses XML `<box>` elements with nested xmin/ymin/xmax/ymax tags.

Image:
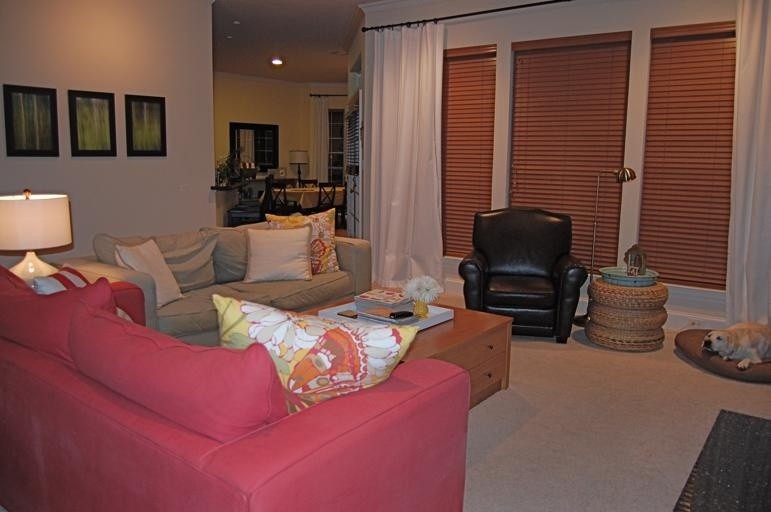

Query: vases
<box><xmin>410</xmin><ymin>298</ymin><xmax>430</xmax><ymax>319</ymax></box>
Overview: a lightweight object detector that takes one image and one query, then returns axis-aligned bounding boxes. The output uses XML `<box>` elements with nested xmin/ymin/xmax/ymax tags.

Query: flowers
<box><xmin>405</xmin><ymin>275</ymin><xmax>443</xmax><ymax>303</ymax></box>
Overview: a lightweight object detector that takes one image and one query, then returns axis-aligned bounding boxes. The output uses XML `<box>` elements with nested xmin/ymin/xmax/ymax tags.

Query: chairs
<box><xmin>262</xmin><ymin>173</ymin><xmax>347</xmax><ymax>229</ymax></box>
<box><xmin>458</xmin><ymin>205</ymin><xmax>588</xmax><ymax>343</ymax></box>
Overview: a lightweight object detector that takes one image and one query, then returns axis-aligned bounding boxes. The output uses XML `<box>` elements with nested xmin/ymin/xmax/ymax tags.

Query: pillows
<box><xmin>264</xmin><ymin>206</ymin><xmax>340</xmax><ymax>273</ymax></box>
<box><xmin>239</xmin><ymin>222</ymin><xmax>313</xmax><ymax>284</ymax></box>
<box><xmin>211</xmin><ymin>291</ymin><xmax>419</xmax><ymax>412</ymax></box>
<box><xmin>113</xmin><ymin>238</ymin><xmax>181</xmax><ymax>309</ymax></box>
<box><xmin>33</xmin><ymin>262</ymin><xmax>132</xmax><ymax>319</ymax></box>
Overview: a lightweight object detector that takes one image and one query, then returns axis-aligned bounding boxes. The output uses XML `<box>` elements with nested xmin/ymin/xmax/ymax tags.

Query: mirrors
<box><xmin>229</xmin><ymin>119</ymin><xmax>278</xmax><ymax>172</ymax></box>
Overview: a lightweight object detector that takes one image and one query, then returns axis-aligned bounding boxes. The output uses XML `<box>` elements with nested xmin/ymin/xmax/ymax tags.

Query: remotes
<box><xmin>390</xmin><ymin>311</ymin><xmax>413</xmax><ymax>319</ymax></box>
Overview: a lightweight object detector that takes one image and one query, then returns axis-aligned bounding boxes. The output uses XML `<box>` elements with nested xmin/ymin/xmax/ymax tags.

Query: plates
<box><xmin>598</xmin><ymin>266</ymin><xmax>659</xmax><ymax>287</ymax></box>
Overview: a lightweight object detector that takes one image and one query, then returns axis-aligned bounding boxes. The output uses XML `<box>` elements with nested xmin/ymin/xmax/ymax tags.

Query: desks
<box><xmin>584</xmin><ymin>276</ymin><xmax>668</xmax><ymax>352</ymax></box>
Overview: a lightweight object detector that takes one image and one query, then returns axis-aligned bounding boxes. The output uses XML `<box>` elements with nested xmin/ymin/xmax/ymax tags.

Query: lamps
<box><xmin>288</xmin><ymin>149</ymin><xmax>308</xmax><ymax>188</ymax></box>
<box><xmin>0</xmin><ymin>184</ymin><xmax>72</xmax><ymax>283</ymax></box>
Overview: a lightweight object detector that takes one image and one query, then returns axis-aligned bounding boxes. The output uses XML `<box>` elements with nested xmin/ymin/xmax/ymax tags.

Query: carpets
<box><xmin>674</xmin><ymin>409</ymin><xmax>770</xmax><ymax>512</ymax></box>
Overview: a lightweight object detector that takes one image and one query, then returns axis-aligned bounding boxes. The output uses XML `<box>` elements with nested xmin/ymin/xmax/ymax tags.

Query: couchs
<box><xmin>0</xmin><ymin>265</ymin><xmax>470</xmax><ymax>512</ymax></box>
<box><xmin>65</xmin><ymin>204</ymin><xmax>375</xmax><ymax>352</ymax></box>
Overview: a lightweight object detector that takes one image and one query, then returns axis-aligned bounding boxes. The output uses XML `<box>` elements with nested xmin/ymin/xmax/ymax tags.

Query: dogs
<box><xmin>701</xmin><ymin>322</ymin><xmax>771</xmax><ymax>370</ymax></box>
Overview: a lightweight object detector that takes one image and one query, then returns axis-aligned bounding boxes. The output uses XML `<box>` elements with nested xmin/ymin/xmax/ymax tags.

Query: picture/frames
<box><xmin>2</xmin><ymin>83</ymin><xmax>59</xmax><ymax>158</ymax></box>
<box><xmin>67</xmin><ymin>88</ymin><xmax>116</xmax><ymax>156</ymax></box>
<box><xmin>124</xmin><ymin>94</ymin><xmax>166</xmax><ymax>157</ymax></box>
